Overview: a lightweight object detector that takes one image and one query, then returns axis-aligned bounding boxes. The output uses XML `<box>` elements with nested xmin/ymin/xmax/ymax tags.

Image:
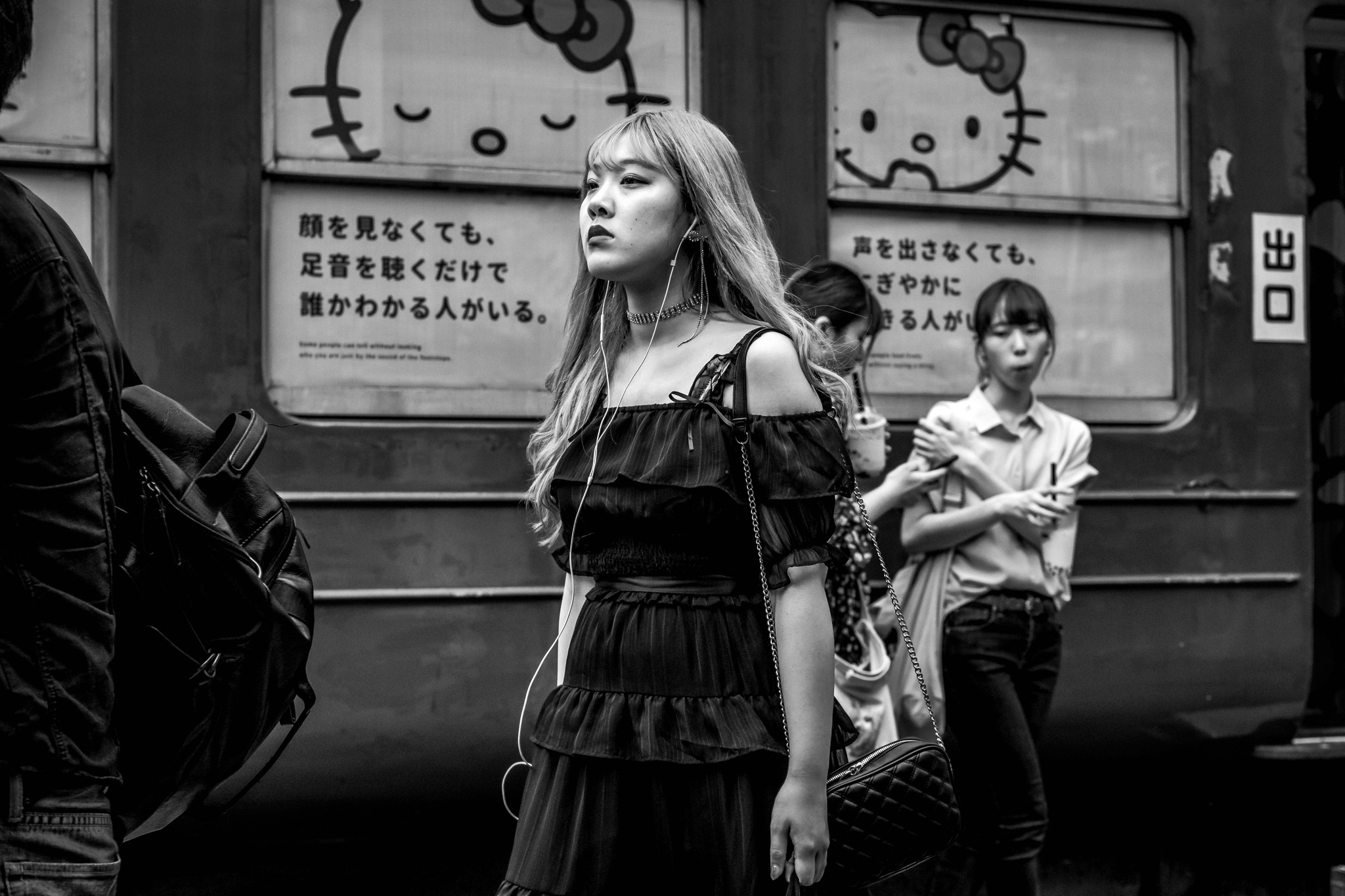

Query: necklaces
<box><xmin>626</xmin><ymin>290</ymin><xmax>709</xmax><ymax>324</ymax></box>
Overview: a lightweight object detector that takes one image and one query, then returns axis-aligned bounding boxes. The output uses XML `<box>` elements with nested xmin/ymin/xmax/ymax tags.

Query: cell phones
<box><xmin>929</xmin><ymin>453</ymin><xmax>958</xmax><ymax>471</ymax></box>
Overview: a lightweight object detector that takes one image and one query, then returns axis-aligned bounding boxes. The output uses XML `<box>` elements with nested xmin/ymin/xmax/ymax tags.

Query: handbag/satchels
<box><xmin>789</xmin><ymin>739</ymin><xmax>963</xmax><ymax>896</ymax></box>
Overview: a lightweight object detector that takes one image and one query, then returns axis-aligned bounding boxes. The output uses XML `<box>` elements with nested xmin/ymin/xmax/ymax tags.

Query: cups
<box><xmin>846</xmin><ymin>410</ymin><xmax>886</xmax><ymax>481</ymax></box>
<box><xmin>1042</xmin><ymin>505</ymin><xmax>1080</xmax><ymax>576</ymax></box>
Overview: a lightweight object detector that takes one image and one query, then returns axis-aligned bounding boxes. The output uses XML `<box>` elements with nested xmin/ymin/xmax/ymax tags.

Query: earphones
<box><xmin>683</xmin><ymin>215</ymin><xmax>702</xmax><ymax>239</ymax></box>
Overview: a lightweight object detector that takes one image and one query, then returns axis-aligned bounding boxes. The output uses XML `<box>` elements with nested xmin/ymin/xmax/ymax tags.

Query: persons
<box><xmin>782</xmin><ymin>260</ymin><xmax>946</xmax><ymax>770</ymax></box>
<box><xmin>498</xmin><ymin>108</ymin><xmax>856</xmax><ymax>896</ymax></box>
<box><xmin>900</xmin><ymin>278</ymin><xmax>1098</xmax><ymax>896</ymax></box>
<box><xmin>1</xmin><ymin>1</ymin><xmax>180</xmax><ymax>895</ymax></box>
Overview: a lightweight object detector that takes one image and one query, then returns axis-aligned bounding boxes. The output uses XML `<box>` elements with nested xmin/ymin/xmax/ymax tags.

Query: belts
<box><xmin>978</xmin><ymin>595</ymin><xmax>1056</xmax><ymax>619</ymax></box>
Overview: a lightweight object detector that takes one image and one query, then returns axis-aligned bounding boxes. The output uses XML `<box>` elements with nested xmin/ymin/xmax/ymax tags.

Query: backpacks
<box><xmin>0</xmin><ymin>174</ymin><xmax>316</xmax><ymax>852</ymax></box>
